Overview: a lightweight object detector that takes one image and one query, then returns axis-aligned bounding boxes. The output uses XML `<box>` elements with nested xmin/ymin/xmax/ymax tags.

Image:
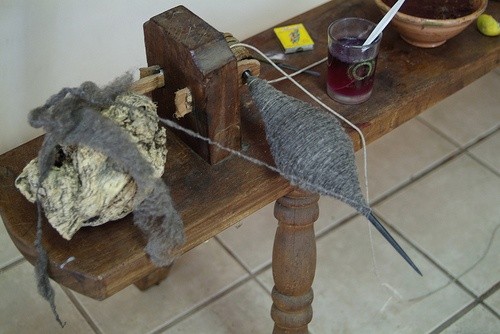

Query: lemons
<box><xmin>476</xmin><ymin>14</ymin><xmax>500</xmax><ymax>36</ymax></box>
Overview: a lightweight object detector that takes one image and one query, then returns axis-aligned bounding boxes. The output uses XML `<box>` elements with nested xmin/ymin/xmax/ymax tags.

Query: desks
<box><xmin>0</xmin><ymin>0</ymin><xmax>500</xmax><ymax>334</ymax></box>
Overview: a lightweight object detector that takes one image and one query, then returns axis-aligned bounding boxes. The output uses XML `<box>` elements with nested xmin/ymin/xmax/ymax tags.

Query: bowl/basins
<box><xmin>375</xmin><ymin>0</ymin><xmax>488</xmax><ymax>47</ymax></box>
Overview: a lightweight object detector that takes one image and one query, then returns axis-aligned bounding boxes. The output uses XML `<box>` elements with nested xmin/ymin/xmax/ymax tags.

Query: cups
<box><xmin>327</xmin><ymin>18</ymin><xmax>383</xmax><ymax>104</ymax></box>
